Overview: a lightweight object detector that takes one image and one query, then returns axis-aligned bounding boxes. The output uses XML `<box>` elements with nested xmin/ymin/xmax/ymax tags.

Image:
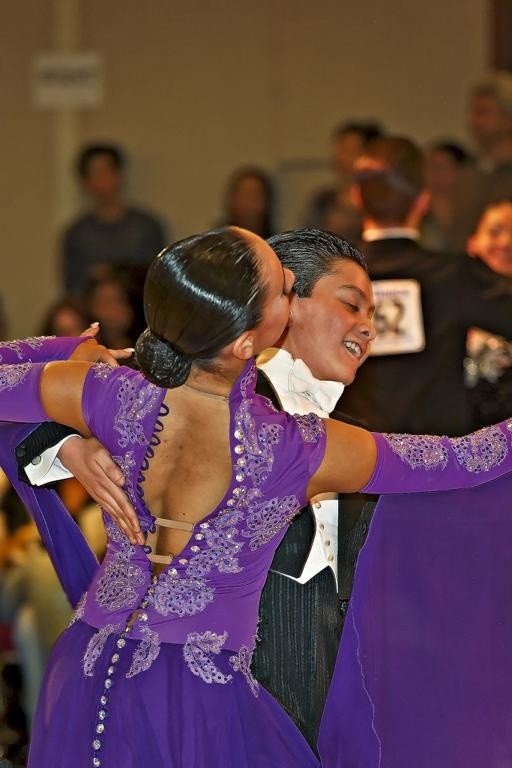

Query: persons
<box><xmin>16</xmin><ymin>229</ymin><xmax>376</xmax><ymax>757</ymax></box>
<box><xmin>465</xmin><ymin>197</ymin><xmax>512</xmax><ymax>430</ymax></box>
<box><xmin>334</xmin><ymin>136</ymin><xmax>512</xmax><ymax>435</ymax></box>
<box><xmin>225</xmin><ymin>169</ymin><xmax>274</xmax><ymax>241</ymax></box>
<box><xmin>1</xmin><ymin>226</ymin><xmax>512</xmax><ymax>768</ymax></box>
<box><xmin>43</xmin><ymin>144</ymin><xmax>165</xmax><ymax>368</ymax></box>
<box><xmin>309</xmin><ymin>72</ymin><xmax>512</xmax><ymax>249</ymax></box>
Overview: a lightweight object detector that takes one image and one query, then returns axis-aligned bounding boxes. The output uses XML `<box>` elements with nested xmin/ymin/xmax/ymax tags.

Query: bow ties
<box><xmin>288</xmin><ymin>359</ymin><xmax>344</xmax><ymax>414</ymax></box>
